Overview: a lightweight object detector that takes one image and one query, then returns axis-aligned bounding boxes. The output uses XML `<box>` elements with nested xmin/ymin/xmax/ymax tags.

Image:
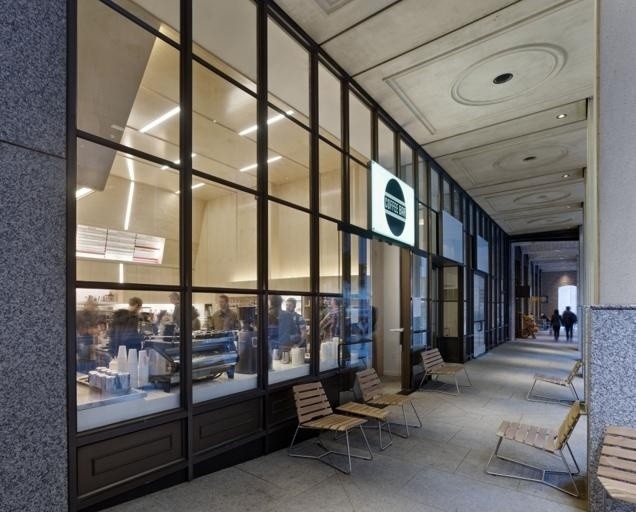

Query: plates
<box><xmin>291</xmin><ymin>347</ymin><xmax>305</xmax><ymax>365</ymax></box>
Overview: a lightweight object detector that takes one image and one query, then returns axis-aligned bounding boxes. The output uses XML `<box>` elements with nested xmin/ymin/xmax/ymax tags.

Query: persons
<box><xmin>539</xmin><ymin>312</ymin><xmax>551</xmax><ymax>330</ymax></box>
<box><xmin>76</xmin><ymin>295</ymin><xmax>170</xmax><ymax>370</ymax></box>
<box><xmin>562</xmin><ymin>305</ymin><xmax>578</xmax><ymax>340</ymax></box>
<box><xmin>550</xmin><ymin>309</ymin><xmax>565</xmax><ymax>340</ymax></box>
<box><xmin>169</xmin><ymin>293</ymin><xmax>241</xmax><ymax>379</ymax></box>
<box><xmin>252</xmin><ymin>294</ymin><xmax>378</xmax><ymax>360</ymax></box>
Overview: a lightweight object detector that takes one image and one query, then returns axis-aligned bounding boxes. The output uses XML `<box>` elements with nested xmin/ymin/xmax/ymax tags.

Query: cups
<box><xmin>319</xmin><ymin>337</ymin><xmax>342</xmax><ymax>363</ymax></box>
<box><xmin>88</xmin><ymin>345</ymin><xmax>149</xmax><ymax>391</ymax></box>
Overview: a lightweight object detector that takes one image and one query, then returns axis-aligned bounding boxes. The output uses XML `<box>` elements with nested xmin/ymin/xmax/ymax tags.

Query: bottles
<box><xmin>281</xmin><ymin>347</ymin><xmax>290</xmax><ymax>364</ymax></box>
<box><xmin>271</xmin><ymin>345</ymin><xmax>281</xmax><ymax>360</ymax></box>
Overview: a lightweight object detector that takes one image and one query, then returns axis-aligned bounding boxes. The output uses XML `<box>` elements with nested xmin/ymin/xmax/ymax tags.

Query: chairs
<box><xmin>418</xmin><ymin>348</ymin><xmax>472</xmax><ymax>396</ymax></box>
<box><xmin>596</xmin><ymin>425</ymin><xmax>636</xmax><ymax>512</ymax></box>
<box><xmin>484</xmin><ymin>359</ymin><xmax>587</xmax><ymax>499</ymax></box>
<box><xmin>286</xmin><ymin>368</ymin><xmax>423</xmax><ymax>476</ymax></box>
